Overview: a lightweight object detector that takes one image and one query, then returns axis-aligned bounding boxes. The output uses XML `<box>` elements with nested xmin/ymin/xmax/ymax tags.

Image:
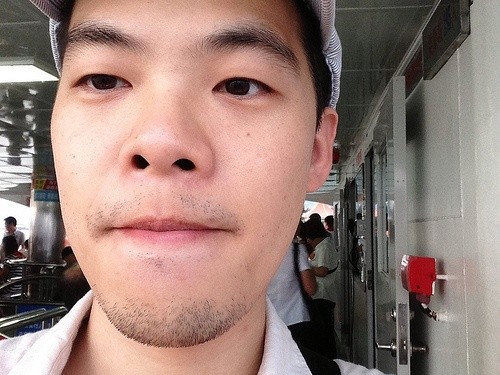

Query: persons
<box><xmin>0</xmin><ymin>0</ymin><xmax>386</xmax><ymax>375</ymax></box>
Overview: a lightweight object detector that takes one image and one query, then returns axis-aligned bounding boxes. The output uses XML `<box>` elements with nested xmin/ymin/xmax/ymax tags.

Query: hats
<box><xmin>60</xmin><ymin>247</ymin><xmax>73</xmax><ymax>260</ymax></box>
<box><xmin>31</xmin><ymin>0</ymin><xmax>341</xmax><ymax>112</ymax></box>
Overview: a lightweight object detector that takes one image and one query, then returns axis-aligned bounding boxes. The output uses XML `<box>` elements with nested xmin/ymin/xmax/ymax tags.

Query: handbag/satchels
<box><xmin>308</xmin><ymin>297</ymin><xmax>338</xmax><ymax>358</ymax></box>
